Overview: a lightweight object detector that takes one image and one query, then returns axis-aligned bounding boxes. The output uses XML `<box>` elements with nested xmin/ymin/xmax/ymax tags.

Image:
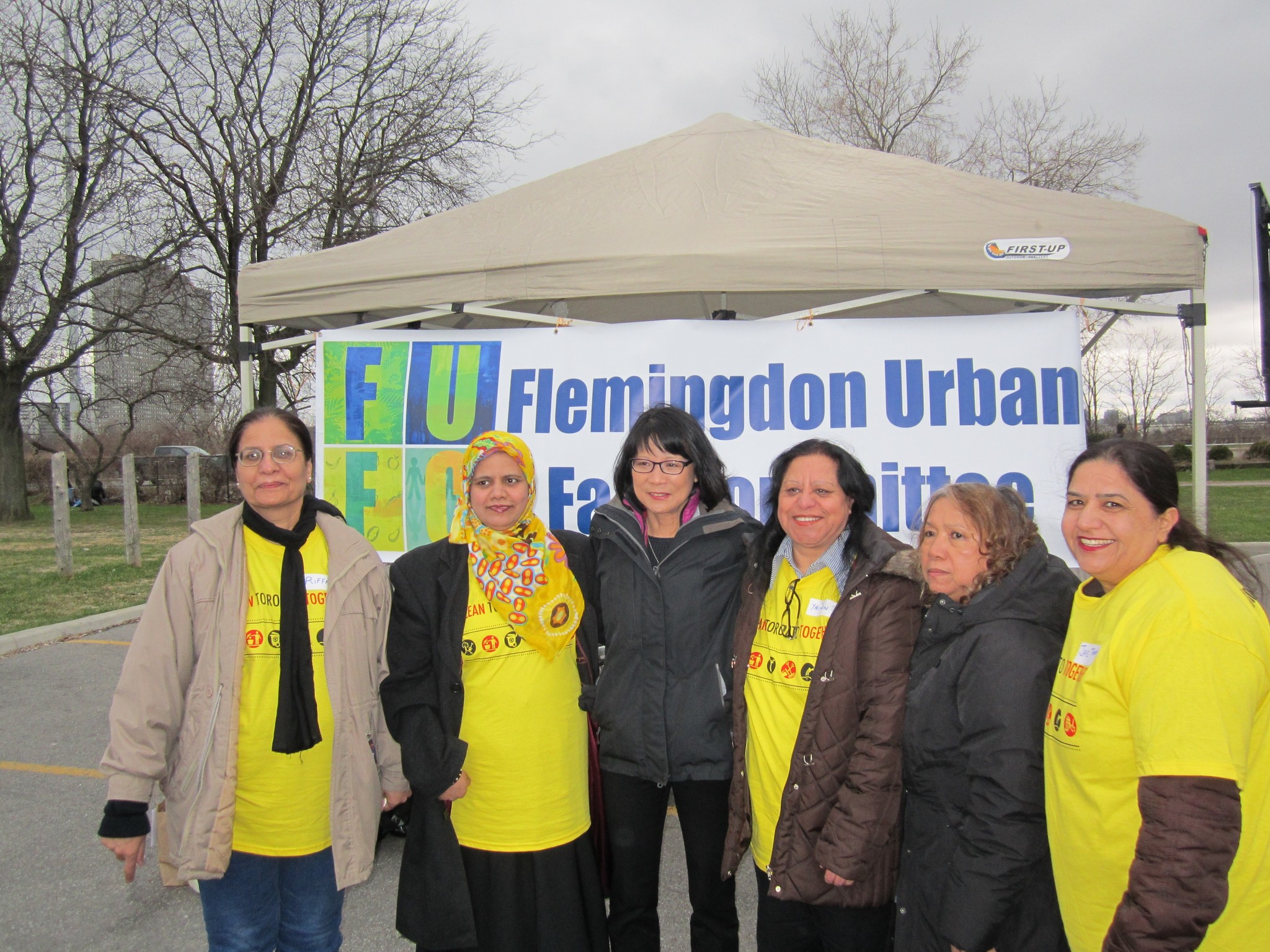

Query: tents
<box><xmin>236</xmin><ymin>112</ymin><xmax>1213</xmax><ymax>568</ymax></box>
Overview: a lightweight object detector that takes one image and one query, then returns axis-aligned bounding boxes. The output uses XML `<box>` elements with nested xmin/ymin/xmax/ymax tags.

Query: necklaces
<box><xmin>646</xmin><ymin>534</ymin><xmax>660</xmax><ymax>564</ymax></box>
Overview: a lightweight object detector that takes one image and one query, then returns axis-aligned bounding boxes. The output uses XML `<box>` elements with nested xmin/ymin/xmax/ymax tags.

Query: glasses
<box><xmin>629</xmin><ymin>458</ymin><xmax>693</xmax><ymax>475</ymax></box>
<box><xmin>235</xmin><ymin>445</ymin><xmax>305</xmax><ymax>467</ymax></box>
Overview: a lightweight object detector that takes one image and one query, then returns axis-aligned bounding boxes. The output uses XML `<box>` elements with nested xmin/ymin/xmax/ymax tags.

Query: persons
<box><xmin>719</xmin><ymin>437</ymin><xmax>938</xmax><ymax>951</ymax></box>
<box><xmin>373</xmin><ymin>427</ymin><xmax>608</xmax><ymax>951</ymax></box>
<box><xmin>584</xmin><ymin>399</ymin><xmax>779</xmax><ymax>952</ymax></box>
<box><xmin>891</xmin><ymin>480</ymin><xmax>1080</xmax><ymax>951</ymax></box>
<box><xmin>1040</xmin><ymin>437</ymin><xmax>1270</xmax><ymax>951</ymax></box>
<box><xmin>96</xmin><ymin>410</ymin><xmax>413</xmax><ymax>952</ymax></box>
